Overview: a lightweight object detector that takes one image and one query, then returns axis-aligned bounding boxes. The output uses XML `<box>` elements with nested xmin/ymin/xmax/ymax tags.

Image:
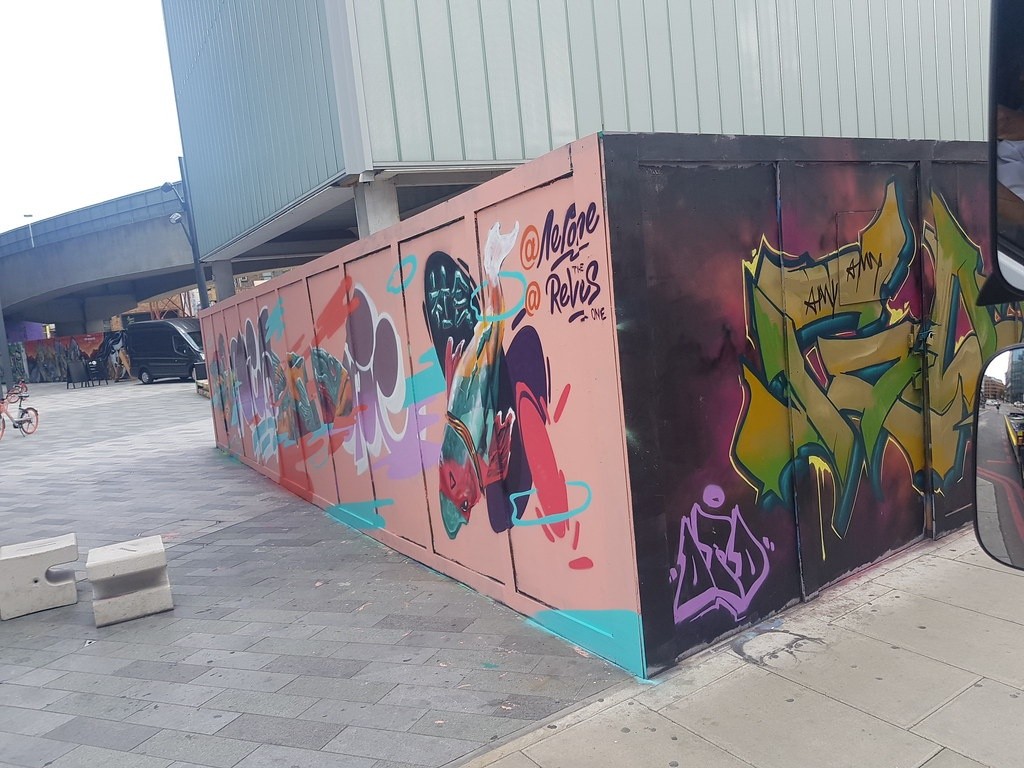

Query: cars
<box><xmin>986</xmin><ymin>400</ymin><xmax>1001</xmax><ymax>406</ymax></box>
<box><xmin>1014</xmin><ymin>402</ymin><xmax>1024</xmax><ymax>408</ymax></box>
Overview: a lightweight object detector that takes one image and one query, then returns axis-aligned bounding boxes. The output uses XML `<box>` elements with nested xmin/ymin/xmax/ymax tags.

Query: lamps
<box><xmin>161</xmin><ymin>182</ymin><xmax>184</xmax><ymax>205</ymax></box>
<box><xmin>169</xmin><ymin>212</ymin><xmax>192</xmax><ymax>246</ymax></box>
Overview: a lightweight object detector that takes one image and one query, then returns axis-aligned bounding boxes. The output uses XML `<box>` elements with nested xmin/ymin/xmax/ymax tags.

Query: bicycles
<box><xmin>6</xmin><ymin>376</ymin><xmax>28</xmax><ymax>404</ymax></box>
<box><xmin>0</xmin><ymin>392</ymin><xmax>39</xmax><ymax>441</ymax></box>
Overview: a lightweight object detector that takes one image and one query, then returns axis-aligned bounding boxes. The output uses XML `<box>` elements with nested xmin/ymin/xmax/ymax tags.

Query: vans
<box><xmin>127</xmin><ymin>318</ymin><xmax>206</xmax><ymax>384</ymax></box>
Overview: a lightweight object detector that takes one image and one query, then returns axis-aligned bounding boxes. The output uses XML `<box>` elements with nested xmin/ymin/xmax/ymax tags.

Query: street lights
<box><xmin>24</xmin><ymin>214</ymin><xmax>35</xmax><ymax>248</ymax></box>
<box><xmin>160</xmin><ymin>156</ymin><xmax>210</xmax><ymax>310</ymax></box>
